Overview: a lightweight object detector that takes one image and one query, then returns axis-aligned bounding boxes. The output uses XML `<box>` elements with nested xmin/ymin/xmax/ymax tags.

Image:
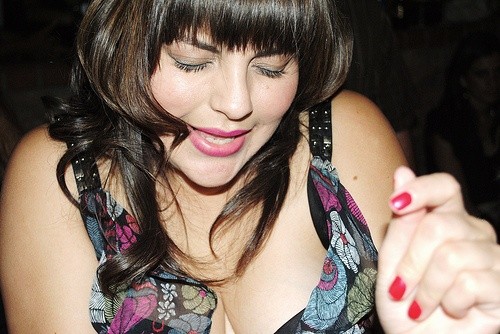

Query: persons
<box><xmin>0</xmin><ymin>0</ymin><xmax>500</xmax><ymax>334</ymax></box>
